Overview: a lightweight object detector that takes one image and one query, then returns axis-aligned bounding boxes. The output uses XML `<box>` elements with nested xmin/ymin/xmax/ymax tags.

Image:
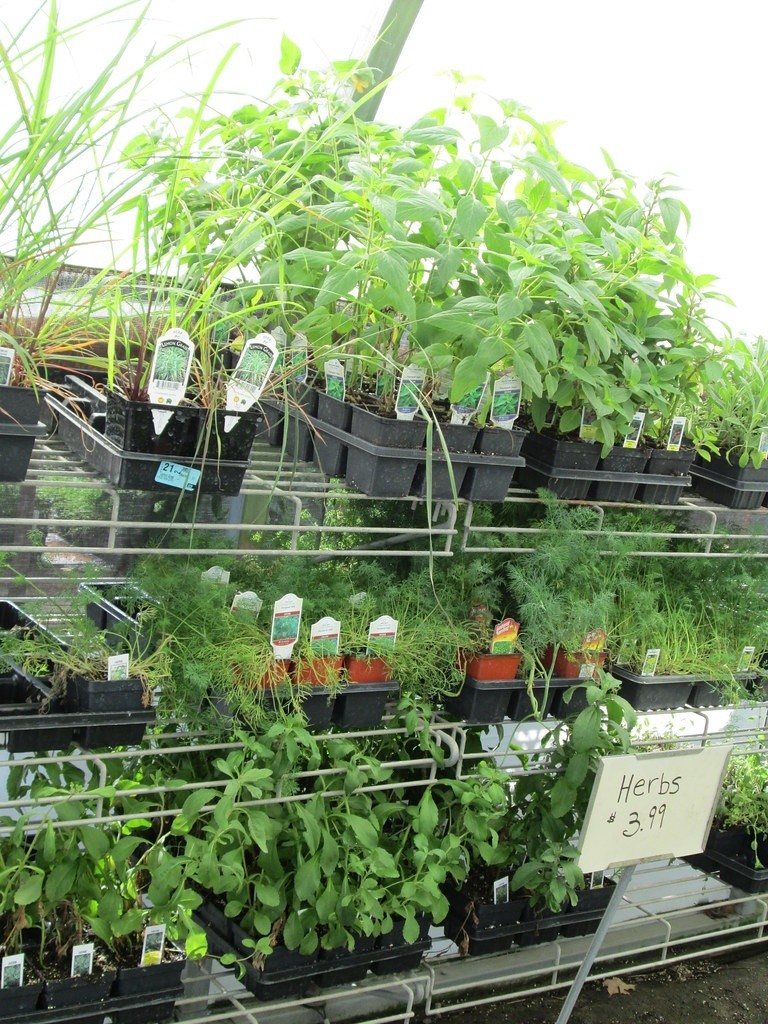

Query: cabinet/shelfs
<box><xmin>1</xmin><ymin>415</ymin><xmax>463</xmax><ymax>1023</ymax></box>
<box><xmin>420</xmin><ymin>485</ymin><xmax>768</xmax><ymax>1016</ymax></box>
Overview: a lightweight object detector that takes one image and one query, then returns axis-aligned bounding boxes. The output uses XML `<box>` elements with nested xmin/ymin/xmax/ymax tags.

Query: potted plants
<box><xmin>1</xmin><ymin>1</ymin><xmax>768</xmax><ymax>1023</ymax></box>
